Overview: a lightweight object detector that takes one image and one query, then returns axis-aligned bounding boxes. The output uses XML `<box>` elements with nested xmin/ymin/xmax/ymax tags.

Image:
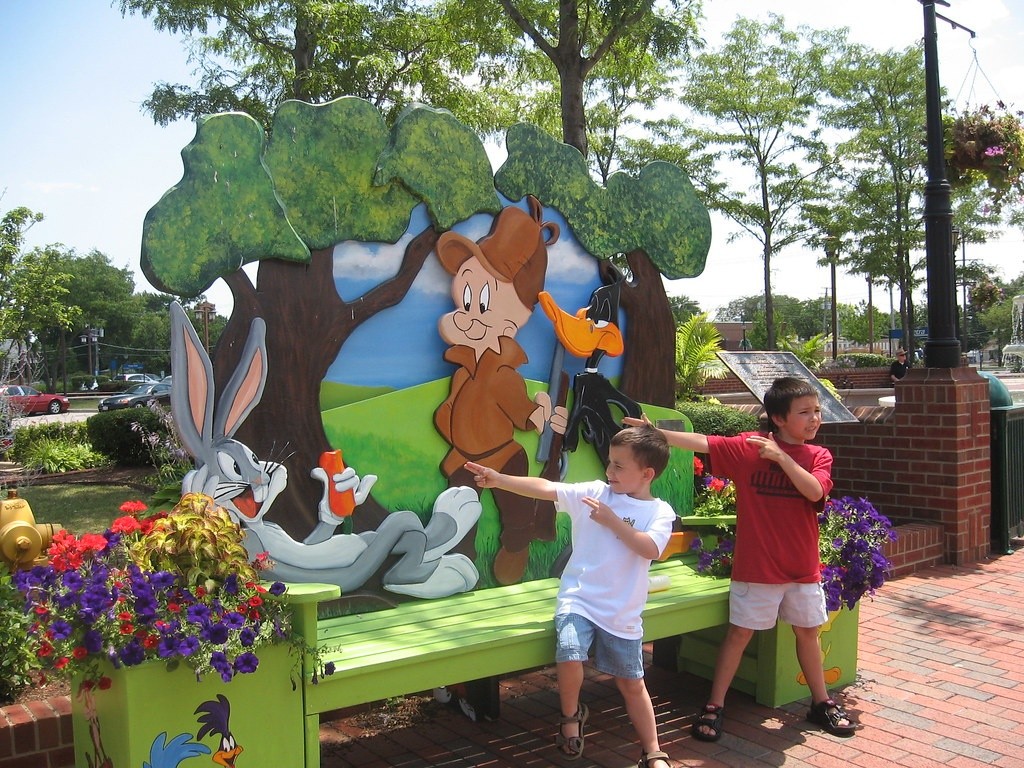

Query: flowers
<box><xmin>685</xmin><ymin>472</ymin><xmax>899</xmax><ymax>611</ymax></box>
<box><xmin>9</xmin><ymin>501</ymin><xmax>340</xmax><ymax>692</ymax></box>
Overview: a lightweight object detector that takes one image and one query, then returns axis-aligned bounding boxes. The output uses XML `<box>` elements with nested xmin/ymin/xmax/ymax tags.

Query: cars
<box><xmin>0</xmin><ymin>385</ymin><xmax>71</xmax><ymax>416</ymax></box>
<box><xmin>114</xmin><ymin>375</ymin><xmax>159</xmax><ymax>385</ymax></box>
<box><xmin>98</xmin><ymin>384</ymin><xmax>171</xmax><ymax>414</ymax></box>
<box><xmin>152</xmin><ymin>376</ymin><xmax>172</xmax><ymax>385</ymax></box>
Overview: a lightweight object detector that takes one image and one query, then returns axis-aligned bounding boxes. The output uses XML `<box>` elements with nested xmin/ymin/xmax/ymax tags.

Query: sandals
<box><xmin>553</xmin><ymin>702</ymin><xmax>590</xmax><ymax>761</ymax></box>
<box><xmin>638</xmin><ymin>748</ymin><xmax>673</xmax><ymax>768</ymax></box>
<box><xmin>806</xmin><ymin>698</ymin><xmax>856</xmax><ymax>734</ymax></box>
<box><xmin>690</xmin><ymin>703</ymin><xmax>728</xmax><ymax>740</ymax></box>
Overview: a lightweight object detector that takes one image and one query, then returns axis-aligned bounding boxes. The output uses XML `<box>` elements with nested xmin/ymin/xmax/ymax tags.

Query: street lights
<box><xmin>81</xmin><ymin>322</ymin><xmax>101</xmax><ymax>376</ymax></box>
<box><xmin>196</xmin><ymin>299</ymin><xmax>217</xmax><ymax>357</ymax></box>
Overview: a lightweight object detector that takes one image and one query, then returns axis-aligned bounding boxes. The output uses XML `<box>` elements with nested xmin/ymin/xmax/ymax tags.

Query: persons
<box><xmin>464</xmin><ymin>424</ymin><xmax>675</xmax><ymax>768</ymax></box>
<box><xmin>621</xmin><ymin>376</ymin><xmax>856</xmax><ymax>741</ymax></box>
<box><xmin>890</xmin><ymin>349</ymin><xmax>912</xmax><ymax>387</ymax></box>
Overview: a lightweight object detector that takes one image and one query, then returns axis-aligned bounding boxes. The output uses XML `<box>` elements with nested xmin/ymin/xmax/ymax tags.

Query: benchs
<box><xmin>251</xmin><ymin>513</ymin><xmax>760</xmax><ymax>768</ymax></box>
